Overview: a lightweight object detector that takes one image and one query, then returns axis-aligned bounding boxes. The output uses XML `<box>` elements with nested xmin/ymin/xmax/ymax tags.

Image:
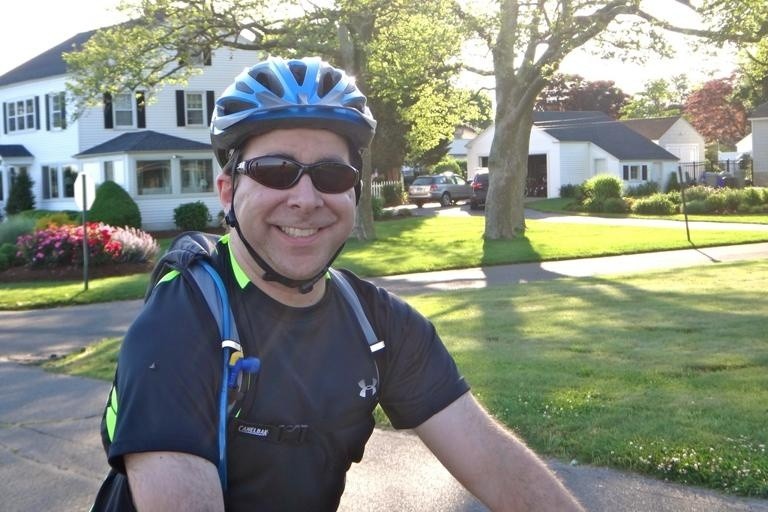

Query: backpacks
<box><xmin>101</xmin><ymin>232</ymin><xmax>385</xmax><ymax>458</ymax></box>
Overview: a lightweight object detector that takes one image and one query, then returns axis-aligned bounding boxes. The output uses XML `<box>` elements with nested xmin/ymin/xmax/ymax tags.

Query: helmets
<box><xmin>210</xmin><ymin>56</ymin><xmax>378</xmax><ymax>169</ymax></box>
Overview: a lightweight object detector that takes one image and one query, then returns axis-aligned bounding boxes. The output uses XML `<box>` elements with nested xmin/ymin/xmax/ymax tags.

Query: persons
<box><xmin>85</xmin><ymin>52</ymin><xmax>583</xmax><ymax>510</ymax></box>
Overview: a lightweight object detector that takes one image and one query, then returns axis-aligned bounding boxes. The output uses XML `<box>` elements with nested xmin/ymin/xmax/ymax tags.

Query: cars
<box><xmin>528</xmin><ymin>173</ymin><xmax>547</xmax><ymax>196</ymax></box>
<box><xmin>700</xmin><ymin>170</ymin><xmax>753</xmax><ymax>188</ymax></box>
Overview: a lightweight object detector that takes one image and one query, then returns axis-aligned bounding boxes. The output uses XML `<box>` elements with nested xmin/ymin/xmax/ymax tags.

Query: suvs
<box><xmin>470</xmin><ymin>170</ymin><xmax>490</xmax><ymax>210</ymax></box>
<box><xmin>407</xmin><ymin>173</ymin><xmax>474</xmax><ymax>208</ymax></box>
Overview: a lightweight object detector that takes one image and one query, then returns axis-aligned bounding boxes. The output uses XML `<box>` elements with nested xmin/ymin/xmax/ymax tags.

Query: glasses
<box><xmin>236</xmin><ymin>154</ymin><xmax>360</xmax><ymax>193</ymax></box>
